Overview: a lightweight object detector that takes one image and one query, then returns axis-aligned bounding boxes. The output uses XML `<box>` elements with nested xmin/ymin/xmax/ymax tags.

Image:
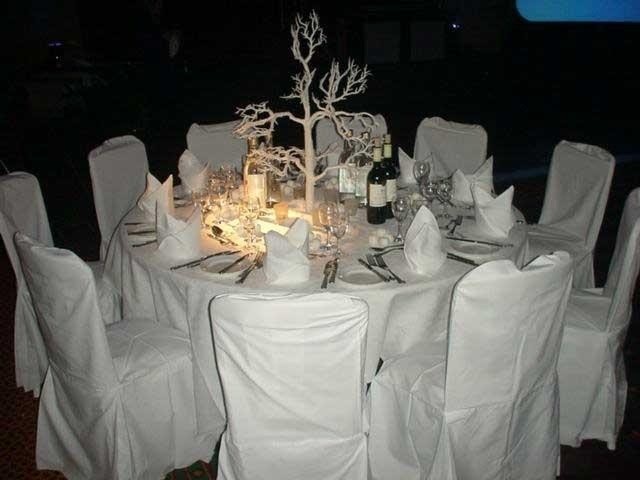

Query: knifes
<box><xmin>169</xmin><ymin>247</ymin><xmax>238</xmax><ymax>271</ymax></box>
<box><xmin>321</xmin><ymin>257</ymin><xmax>339</xmax><ymax>290</ymax></box>
<box><xmin>357</xmin><ymin>258</ymin><xmax>389</xmax><ymax>283</ymax></box>
<box><xmin>219</xmin><ymin>252</ymin><xmax>250</xmax><ymax>275</ymax></box>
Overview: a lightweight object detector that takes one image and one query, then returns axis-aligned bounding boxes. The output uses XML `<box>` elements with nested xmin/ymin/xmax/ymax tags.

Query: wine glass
<box><xmin>412</xmin><ymin>160</ymin><xmax>453</xmax><ymax>221</ymax></box>
<box><xmin>240</xmin><ymin>195</ymin><xmax>261</xmax><ymax>251</ymax></box>
<box><xmin>196</xmin><ymin>163</ymin><xmax>237</xmax><ymax>236</ymax></box>
<box><xmin>319</xmin><ymin>201</ymin><xmax>349</xmax><ymax>259</ymax></box>
<box><xmin>390</xmin><ymin>194</ymin><xmax>410</xmax><ymax>242</ymax></box>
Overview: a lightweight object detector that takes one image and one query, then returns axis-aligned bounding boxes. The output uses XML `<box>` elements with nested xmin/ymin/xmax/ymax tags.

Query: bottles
<box><xmin>241</xmin><ymin>129</ymin><xmax>280</xmax><ymax>208</ymax></box>
<box><xmin>338</xmin><ymin>128</ymin><xmax>371</xmax><ymax>208</ymax></box>
<box><xmin>366</xmin><ymin>141</ymin><xmax>397</xmax><ymax>225</ymax></box>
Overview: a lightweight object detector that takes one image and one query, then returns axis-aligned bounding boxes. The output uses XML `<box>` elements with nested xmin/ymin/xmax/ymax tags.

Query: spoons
<box><xmin>369</xmin><ymin>244</ymin><xmax>406</xmax><ymax>251</ymax></box>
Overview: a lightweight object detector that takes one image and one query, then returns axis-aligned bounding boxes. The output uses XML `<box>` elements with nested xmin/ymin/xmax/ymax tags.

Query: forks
<box><xmin>366</xmin><ymin>252</ymin><xmax>406</xmax><ymax>286</ymax></box>
<box><xmin>448</xmin><ymin>215</ymin><xmax>464</xmax><ymax>236</ymax></box>
<box><xmin>236</xmin><ymin>250</ymin><xmax>266</xmax><ymax>286</ymax></box>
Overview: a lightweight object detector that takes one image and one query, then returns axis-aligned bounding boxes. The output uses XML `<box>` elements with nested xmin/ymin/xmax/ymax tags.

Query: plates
<box><xmin>445</xmin><ymin>234</ymin><xmax>508</xmax><ymax>259</ymax></box>
<box><xmin>200</xmin><ymin>254</ymin><xmax>250</xmax><ymax>276</ymax></box>
<box><xmin>337</xmin><ymin>263</ymin><xmax>392</xmax><ymax>287</ymax></box>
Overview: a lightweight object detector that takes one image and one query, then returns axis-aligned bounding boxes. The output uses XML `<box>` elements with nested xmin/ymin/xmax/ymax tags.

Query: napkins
<box><xmin>137</xmin><ymin>172</ymin><xmax>175</xmax><ymax>217</ymax></box>
<box><xmin>452</xmin><ymin>154</ymin><xmax>494</xmax><ymax>203</ymax></box>
<box><xmin>178</xmin><ymin>151</ymin><xmax>209</xmax><ymax>194</ymax></box>
<box><xmin>469</xmin><ymin>181</ymin><xmax>516</xmax><ymax>242</ymax></box>
<box><xmin>403</xmin><ymin>203</ymin><xmax>446</xmax><ymax>277</ymax></box>
<box><xmin>397</xmin><ymin>148</ymin><xmax>419</xmax><ymax>184</ymax></box>
<box><xmin>258</xmin><ymin>216</ymin><xmax>311</xmax><ymax>287</ymax></box>
<box><xmin>157</xmin><ymin>203</ymin><xmax>204</xmax><ymax>262</ymax></box>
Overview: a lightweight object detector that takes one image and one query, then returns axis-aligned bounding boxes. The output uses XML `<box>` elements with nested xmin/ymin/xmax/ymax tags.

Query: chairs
<box><xmin>414</xmin><ymin>115</ymin><xmax>489</xmax><ymax>175</ymax></box>
<box><xmin>525</xmin><ymin>139</ymin><xmax>617</xmax><ymax>287</ymax></box>
<box><xmin>88</xmin><ymin>134</ymin><xmax>149</xmax><ymax>241</ymax></box>
<box><xmin>1</xmin><ymin>170</ymin><xmax>119</xmax><ymax>399</ymax></box>
<box><xmin>557</xmin><ymin>187</ymin><xmax>634</xmax><ymax>450</ymax></box>
<box><xmin>366</xmin><ymin>251</ymin><xmax>575</xmax><ymax>480</ymax></box>
<box><xmin>187</xmin><ymin>118</ymin><xmax>251</xmax><ymax>173</ymax></box>
<box><xmin>315</xmin><ymin>115</ymin><xmax>387</xmax><ymax>150</ymax></box>
<box><xmin>13</xmin><ymin>230</ymin><xmax>225</xmax><ymax>480</ymax></box>
<box><xmin>207</xmin><ymin>289</ymin><xmax>372</xmax><ymax>480</ymax></box>
